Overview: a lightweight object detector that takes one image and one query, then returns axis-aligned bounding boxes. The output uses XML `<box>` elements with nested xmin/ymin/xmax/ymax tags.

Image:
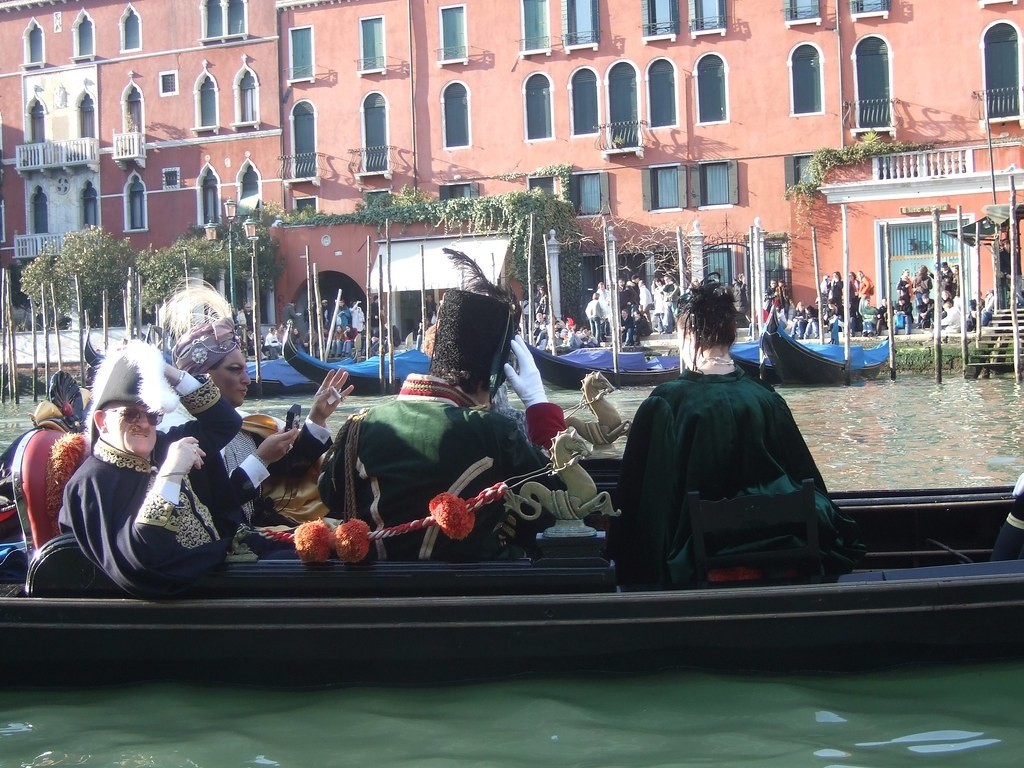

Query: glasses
<box><xmin>539</xmin><ymin>289</ymin><xmax>543</xmax><ymax>290</ymax></box>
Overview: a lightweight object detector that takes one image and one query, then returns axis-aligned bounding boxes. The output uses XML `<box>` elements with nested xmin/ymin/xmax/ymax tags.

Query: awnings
<box><xmin>370</xmin><ymin>234</ymin><xmax>512</xmax><ymax>293</ymax></box>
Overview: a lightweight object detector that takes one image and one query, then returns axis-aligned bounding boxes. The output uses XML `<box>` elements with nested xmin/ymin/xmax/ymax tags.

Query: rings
<box><xmin>194</xmin><ymin>449</ymin><xmax>197</xmax><ymax>455</ymax></box>
<box><xmin>287</xmin><ymin>443</ymin><xmax>293</xmax><ymax>453</ymax></box>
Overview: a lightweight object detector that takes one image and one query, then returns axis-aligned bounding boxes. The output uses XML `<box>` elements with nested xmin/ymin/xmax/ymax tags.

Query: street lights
<box><xmin>203</xmin><ymin>198</ymin><xmax>259</xmax><ymax>324</ymax></box>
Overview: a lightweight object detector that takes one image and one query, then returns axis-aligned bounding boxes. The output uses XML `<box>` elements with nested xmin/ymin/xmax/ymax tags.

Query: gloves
<box><xmin>503</xmin><ymin>335</ymin><xmax>549</xmax><ymax>409</ymax></box>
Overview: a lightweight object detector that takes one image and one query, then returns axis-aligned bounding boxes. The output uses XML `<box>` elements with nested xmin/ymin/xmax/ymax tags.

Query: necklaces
<box><xmin>699</xmin><ymin>355</ymin><xmax>734</xmax><ymax>365</ymax></box>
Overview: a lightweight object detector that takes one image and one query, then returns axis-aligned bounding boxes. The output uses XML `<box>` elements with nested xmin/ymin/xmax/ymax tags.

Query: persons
<box><xmin>607</xmin><ymin>279</ymin><xmax>867</xmax><ymax>591</ymax></box>
<box><xmin>566</xmin><ymin>275</ymin><xmax>679</xmax><ymax>346</ymax></box>
<box><xmin>731</xmin><ymin>272</ymin><xmax>751</xmax><ymax>322</ymax></box>
<box><xmin>58</xmin><ymin>278</ymin><xmax>569</xmax><ymax>599</ymax></box>
<box><xmin>766</xmin><ymin>262</ymin><xmax>995</xmax><ymax>340</ymax></box>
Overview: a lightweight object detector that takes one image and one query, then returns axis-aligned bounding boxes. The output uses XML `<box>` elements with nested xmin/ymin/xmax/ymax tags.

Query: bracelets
<box><xmin>175</xmin><ymin>369</ymin><xmax>185</xmax><ymax>385</ymax></box>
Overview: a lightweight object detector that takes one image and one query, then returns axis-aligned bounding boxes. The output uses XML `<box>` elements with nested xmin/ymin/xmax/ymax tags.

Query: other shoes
<box><xmin>862</xmin><ymin>330</ymin><xmax>874</xmax><ymax>337</ymax></box>
<box><xmin>535</xmin><ymin>343</ymin><xmax>539</xmax><ymax>347</ymax></box>
<box><xmin>624</xmin><ymin>341</ymin><xmax>640</xmax><ymax>346</ymax></box>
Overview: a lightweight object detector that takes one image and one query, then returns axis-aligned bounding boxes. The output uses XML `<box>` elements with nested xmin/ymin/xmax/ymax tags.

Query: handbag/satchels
<box><xmin>869</xmin><ymin>281</ymin><xmax>875</xmax><ymax>295</ymax></box>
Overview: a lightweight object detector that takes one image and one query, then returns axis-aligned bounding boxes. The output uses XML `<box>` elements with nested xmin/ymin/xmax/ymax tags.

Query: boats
<box><xmin>827</xmin><ymin>487</ymin><xmax>1024</xmax><ymax>568</ymax></box>
<box><xmin>761</xmin><ymin>302</ymin><xmax>890</xmax><ymax>389</ymax></box>
<box><xmin>281</xmin><ymin>318</ymin><xmax>434</xmax><ymax>397</ymax></box>
<box><xmin>730</xmin><ymin>339</ymin><xmax>777</xmax><ymax>386</ymax></box>
<box><xmin>84</xmin><ymin>326</ymin><xmax>173</xmax><ymax>395</ymax></box>
<box><xmin>0</xmin><ymin>559</ymin><xmax>1022</xmax><ymax>692</ymax></box>
<box><xmin>246</xmin><ymin>356</ymin><xmax>355</xmax><ymax>399</ymax></box>
<box><xmin>521</xmin><ymin>339</ymin><xmax>679</xmax><ymax>392</ymax></box>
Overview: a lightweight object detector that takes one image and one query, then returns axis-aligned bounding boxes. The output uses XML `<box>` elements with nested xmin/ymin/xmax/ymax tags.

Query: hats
<box><xmin>428</xmin><ymin>247</ymin><xmax>523</xmax><ymax>396</ymax></box>
<box><xmin>88</xmin><ymin>338</ymin><xmax>180</xmax><ymax>453</ymax></box>
<box><xmin>990</xmin><ymin>290</ymin><xmax>994</xmax><ymax>293</ymax></box>
<box><xmin>162</xmin><ymin>276</ymin><xmax>241</xmax><ymax>377</ymax></box>
<box><xmin>941</xmin><ymin>262</ymin><xmax>947</xmax><ymax>265</ymax></box>
